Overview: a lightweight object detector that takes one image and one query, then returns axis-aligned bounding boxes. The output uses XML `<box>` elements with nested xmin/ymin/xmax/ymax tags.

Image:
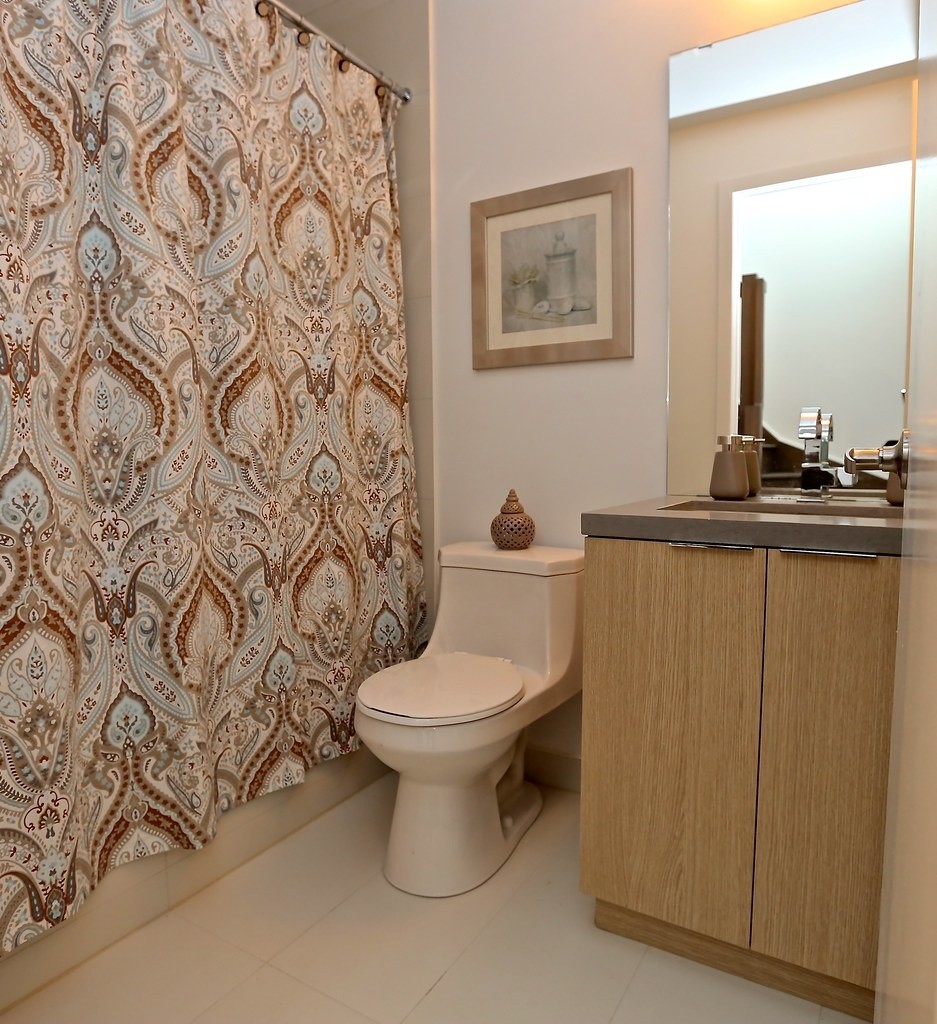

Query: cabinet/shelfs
<box><xmin>578</xmin><ymin>537</ymin><xmax>901</xmax><ymax>1022</ymax></box>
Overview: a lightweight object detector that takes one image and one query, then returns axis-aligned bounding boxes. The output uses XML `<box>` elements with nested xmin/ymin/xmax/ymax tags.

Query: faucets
<box><xmin>796</xmin><ymin>405</ymin><xmax>859</xmax><ymax>504</ymax></box>
<box><xmin>811</xmin><ymin>412</ymin><xmax>859</xmax><ymax>498</ymax></box>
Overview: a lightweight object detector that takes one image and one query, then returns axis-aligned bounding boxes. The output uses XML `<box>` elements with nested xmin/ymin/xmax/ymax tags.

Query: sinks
<box><xmin>655</xmin><ymin>498</ymin><xmax>906</xmax><ymax>521</ymax></box>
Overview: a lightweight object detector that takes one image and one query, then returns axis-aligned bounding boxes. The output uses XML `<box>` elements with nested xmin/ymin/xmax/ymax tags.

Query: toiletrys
<box><xmin>740</xmin><ymin>435</ymin><xmax>767</xmax><ymax>499</ymax></box>
<box><xmin>707</xmin><ymin>433</ymin><xmax>750</xmax><ymax>502</ymax></box>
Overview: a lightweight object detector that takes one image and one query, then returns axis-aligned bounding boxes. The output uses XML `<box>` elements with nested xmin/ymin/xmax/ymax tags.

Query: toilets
<box><xmin>349</xmin><ymin>531</ymin><xmax>593</xmax><ymax>900</ymax></box>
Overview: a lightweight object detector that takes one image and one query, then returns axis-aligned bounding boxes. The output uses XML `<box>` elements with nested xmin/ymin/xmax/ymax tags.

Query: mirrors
<box><xmin>666</xmin><ymin>70</ymin><xmax>917</xmax><ymax>496</ymax></box>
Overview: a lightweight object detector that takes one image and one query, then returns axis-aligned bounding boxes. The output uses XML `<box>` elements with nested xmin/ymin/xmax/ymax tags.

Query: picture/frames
<box><xmin>470</xmin><ymin>167</ymin><xmax>634</xmax><ymax>369</ymax></box>
